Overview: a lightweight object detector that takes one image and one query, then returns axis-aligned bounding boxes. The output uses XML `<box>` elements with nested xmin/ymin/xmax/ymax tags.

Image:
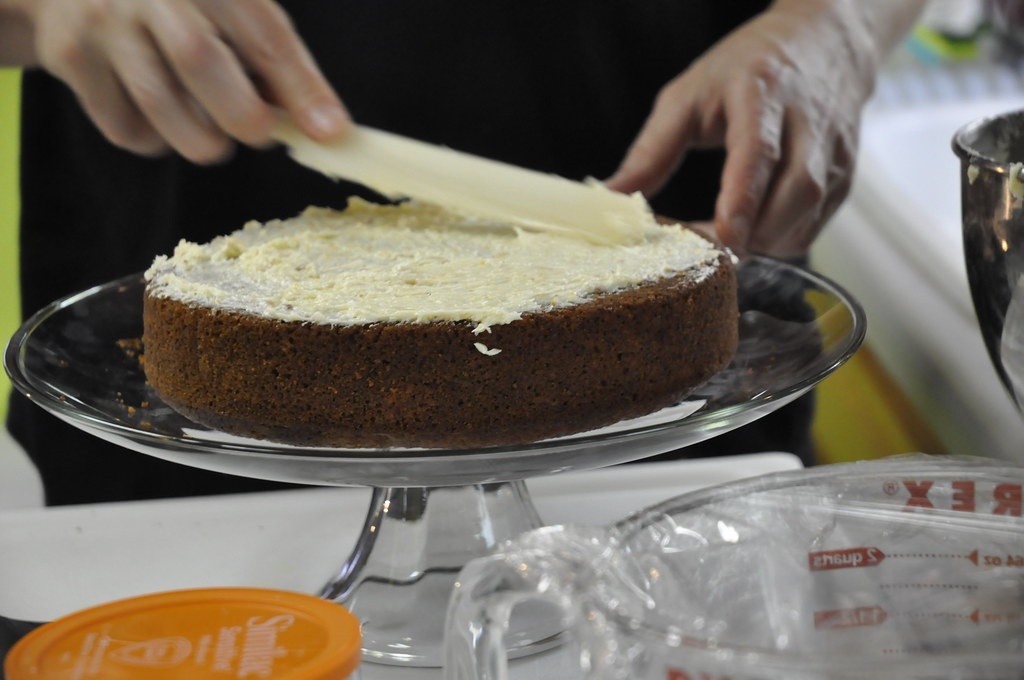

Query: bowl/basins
<box><xmin>951</xmin><ymin>105</ymin><xmax>1024</xmax><ymax>433</ymax></box>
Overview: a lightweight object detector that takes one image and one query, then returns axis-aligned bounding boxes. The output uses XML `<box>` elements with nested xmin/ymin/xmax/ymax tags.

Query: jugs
<box><xmin>443</xmin><ymin>455</ymin><xmax>1021</xmax><ymax>679</ymax></box>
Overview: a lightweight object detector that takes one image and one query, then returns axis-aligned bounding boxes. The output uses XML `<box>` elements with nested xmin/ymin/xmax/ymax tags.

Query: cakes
<box><xmin>144</xmin><ymin>193</ymin><xmax>741</xmax><ymax>451</ymax></box>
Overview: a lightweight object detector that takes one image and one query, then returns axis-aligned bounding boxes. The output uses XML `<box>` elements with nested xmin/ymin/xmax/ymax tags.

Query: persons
<box><xmin>0</xmin><ymin>0</ymin><xmax>925</xmax><ymax>508</ymax></box>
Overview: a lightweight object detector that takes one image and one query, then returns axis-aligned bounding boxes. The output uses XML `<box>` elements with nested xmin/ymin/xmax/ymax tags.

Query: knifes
<box><xmin>230</xmin><ymin>102</ymin><xmax>645</xmax><ymax>249</ymax></box>
